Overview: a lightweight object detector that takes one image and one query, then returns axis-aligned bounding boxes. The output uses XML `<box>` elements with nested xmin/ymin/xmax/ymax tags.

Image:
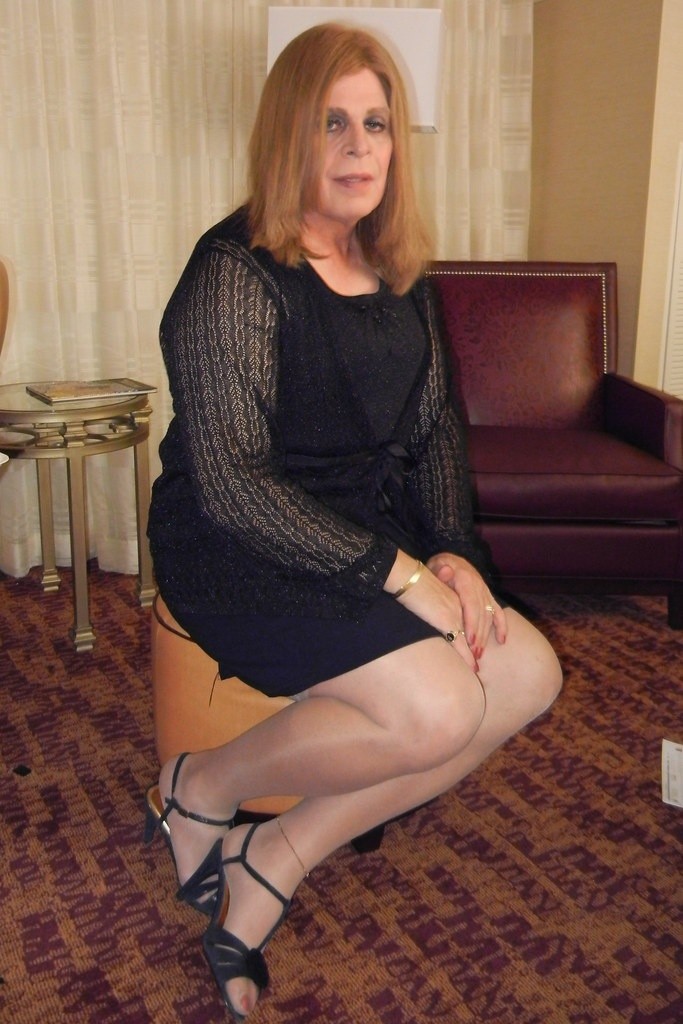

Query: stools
<box><xmin>151</xmin><ymin>591</ymin><xmax>386</xmax><ymax>853</ymax></box>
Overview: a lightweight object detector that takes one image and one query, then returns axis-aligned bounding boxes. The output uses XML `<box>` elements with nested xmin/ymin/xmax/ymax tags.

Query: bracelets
<box><xmin>392</xmin><ymin>561</ymin><xmax>424</xmax><ymax>600</ymax></box>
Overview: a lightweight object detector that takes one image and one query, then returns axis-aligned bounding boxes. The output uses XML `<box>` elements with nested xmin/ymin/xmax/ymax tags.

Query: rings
<box><xmin>485</xmin><ymin>606</ymin><xmax>495</xmax><ymax>615</ymax></box>
<box><xmin>461</xmin><ymin>632</ymin><xmax>465</xmax><ymax>636</ymax></box>
<box><xmin>446</xmin><ymin>631</ymin><xmax>459</xmax><ymax>644</ymax></box>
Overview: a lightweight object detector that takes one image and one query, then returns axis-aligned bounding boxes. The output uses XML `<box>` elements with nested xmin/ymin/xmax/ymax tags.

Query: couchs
<box><xmin>424</xmin><ymin>260</ymin><xmax>683</xmax><ymax>631</ymax></box>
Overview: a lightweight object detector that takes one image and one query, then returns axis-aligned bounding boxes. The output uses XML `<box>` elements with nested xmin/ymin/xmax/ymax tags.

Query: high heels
<box><xmin>144</xmin><ymin>752</ymin><xmax>233</xmax><ymax>916</ymax></box>
<box><xmin>175</xmin><ymin>822</ymin><xmax>291</xmax><ymax>1022</ymax></box>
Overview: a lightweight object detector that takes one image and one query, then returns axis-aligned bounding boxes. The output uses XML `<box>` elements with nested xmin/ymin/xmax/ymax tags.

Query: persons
<box><xmin>145</xmin><ymin>27</ymin><xmax>564</xmax><ymax>1019</ymax></box>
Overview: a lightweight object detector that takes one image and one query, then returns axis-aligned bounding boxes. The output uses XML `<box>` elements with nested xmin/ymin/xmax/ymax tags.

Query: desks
<box><xmin>0</xmin><ymin>381</ymin><xmax>156</xmax><ymax>652</ymax></box>
